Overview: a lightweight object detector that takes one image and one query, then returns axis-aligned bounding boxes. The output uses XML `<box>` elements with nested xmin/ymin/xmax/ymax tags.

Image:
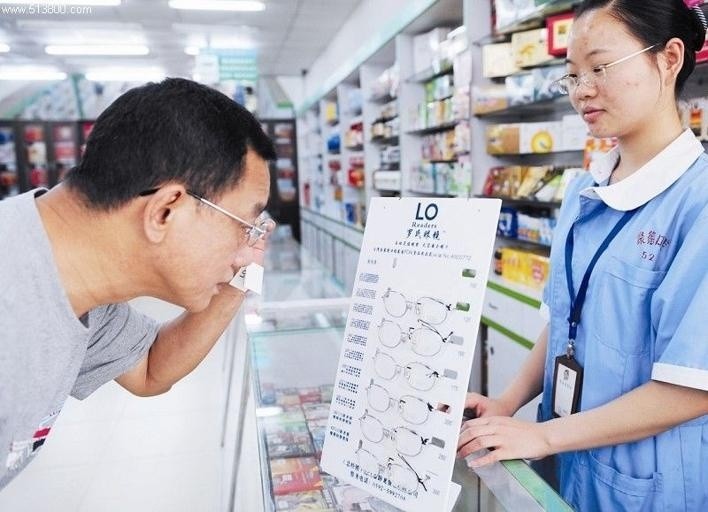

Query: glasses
<box><xmin>354</xmin><ymin>285</ymin><xmax>458</xmax><ymax>495</ymax></box>
<box><xmin>555</xmin><ymin>43</ymin><xmax>660</xmax><ymax>95</ymax></box>
<box><xmin>139</xmin><ymin>188</ymin><xmax>276</xmax><ymax>248</ymax></box>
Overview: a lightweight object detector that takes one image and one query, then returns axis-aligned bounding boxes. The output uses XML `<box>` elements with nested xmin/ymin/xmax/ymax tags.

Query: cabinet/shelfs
<box><xmin>0</xmin><ymin>1</ymin><xmax>706</xmax><ymax>512</ymax></box>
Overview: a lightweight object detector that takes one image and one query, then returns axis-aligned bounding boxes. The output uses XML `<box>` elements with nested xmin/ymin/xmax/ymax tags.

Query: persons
<box><xmin>456</xmin><ymin>0</ymin><xmax>708</xmax><ymax>512</ymax></box>
<box><xmin>0</xmin><ymin>77</ymin><xmax>276</xmax><ymax>491</ymax></box>
